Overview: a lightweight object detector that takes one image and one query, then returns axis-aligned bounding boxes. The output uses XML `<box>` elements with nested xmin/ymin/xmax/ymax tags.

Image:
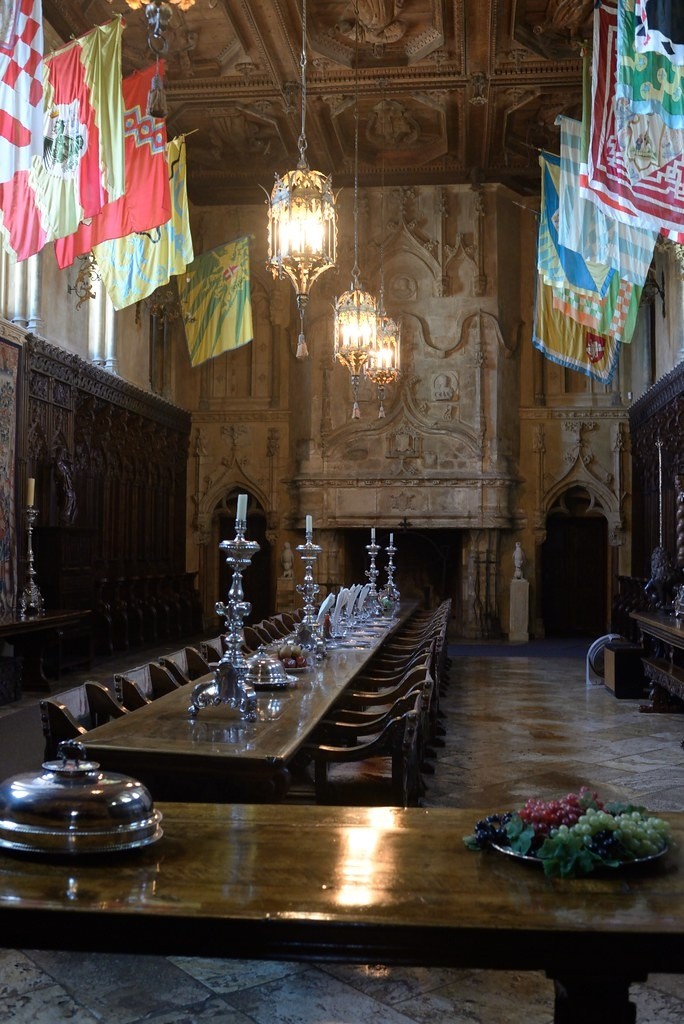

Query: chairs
<box><xmin>39</xmin><ymin>600</ymin><xmax>453</xmax><ymax>809</ymax></box>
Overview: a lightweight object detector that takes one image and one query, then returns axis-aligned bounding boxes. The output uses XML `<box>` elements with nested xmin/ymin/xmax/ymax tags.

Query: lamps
<box><xmin>125</xmin><ymin>0</ymin><xmax>198</xmax><ymax>118</ymax></box>
<box><xmin>258</xmin><ymin>0</ymin><xmax>403</xmax><ymax>421</ymax></box>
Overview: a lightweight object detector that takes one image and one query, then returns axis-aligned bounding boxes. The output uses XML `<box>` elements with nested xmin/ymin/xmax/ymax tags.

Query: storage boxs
<box><xmin>0</xmin><ymin>654</ymin><xmax>24</xmax><ymax>705</ymax></box>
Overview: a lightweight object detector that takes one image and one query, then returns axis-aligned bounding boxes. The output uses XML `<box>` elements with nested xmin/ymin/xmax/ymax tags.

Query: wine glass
<box><xmin>348</xmin><ymin>619</ymin><xmax>357</xmax><ymax>631</ymax></box>
<box><xmin>338</xmin><ymin>623</ymin><xmax>347</xmax><ymax>639</ymax></box>
<box><xmin>356</xmin><ymin>606</ymin><xmax>364</xmax><ymax>625</ymax></box>
<box><xmin>329</xmin><ymin>625</ymin><xmax>338</xmax><ymax>647</ymax></box>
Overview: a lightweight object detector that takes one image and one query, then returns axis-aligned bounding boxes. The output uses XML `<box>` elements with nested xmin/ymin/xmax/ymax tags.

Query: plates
<box><xmin>339</xmin><ymin>641</ymin><xmax>371</xmax><ymax>647</ymax></box>
<box><xmin>366</xmin><ymin>623</ymin><xmax>388</xmax><ymax>627</ymax></box>
<box><xmin>374</xmin><ymin>617</ymin><xmax>392</xmax><ymax>620</ymax></box>
<box><xmin>352</xmin><ymin>631</ymin><xmax>380</xmax><ymax>636</ymax></box>
<box><xmin>284</xmin><ymin>666</ymin><xmax>311</xmax><ymax>673</ymax></box>
<box><xmin>251</xmin><ymin>675</ymin><xmax>299</xmax><ymax>689</ymax></box>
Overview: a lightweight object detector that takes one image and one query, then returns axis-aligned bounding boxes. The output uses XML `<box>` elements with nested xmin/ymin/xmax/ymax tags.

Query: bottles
<box><xmin>322</xmin><ymin>613</ymin><xmax>331</xmax><ymax>638</ymax></box>
<box><xmin>373</xmin><ymin>605</ymin><xmax>382</xmax><ymax>619</ymax></box>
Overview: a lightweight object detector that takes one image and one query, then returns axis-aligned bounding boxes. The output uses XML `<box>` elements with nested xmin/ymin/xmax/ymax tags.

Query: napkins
<box><xmin>334</xmin><ymin>583</ymin><xmax>370</xmax><ymax>620</ymax></box>
<box><xmin>316</xmin><ymin>592</ymin><xmax>335</xmax><ymax>623</ymax></box>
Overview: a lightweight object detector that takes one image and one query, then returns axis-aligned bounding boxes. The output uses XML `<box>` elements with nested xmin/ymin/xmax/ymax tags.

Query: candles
<box><xmin>236</xmin><ymin>494</ymin><xmax>247</xmax><ymax>520</ymax></box>
<box><xmin>370</xmin><ymin>528</ymin><xmax>375</xmax><ymax>539</ymax></box>
<box><xmin>306</xmin><ymin>515</ymin><xmax>312</xmax><ymax>532</ymax></box>
<box><xmin>390</xmin><ymin>532</ymin><xmax>393</xmax><ymax>542</ymax></box>
<box><xmin>27</xmin><ymin>478</ymin><xmax>35</xmax><ymax>506</ymax></box>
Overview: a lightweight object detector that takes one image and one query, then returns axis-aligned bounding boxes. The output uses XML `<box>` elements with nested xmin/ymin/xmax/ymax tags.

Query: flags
<box><xmin>51</xmin><ymin>61</ymin><xmax>171</xmax><ymax>266</ymax></box>
<box><xmin>0</xmin><ymin>22</ymin><xmax>123</xmax><ymax>262</ymax></box>
<box><xmin>613</xmin><ymin>0</ymin><xmax>683</xmax><ymax>130</ymax></box>
<box><xmin>90</xmin><ymin>133</ymin><xmax>195</xmax><ymax>310</ymax></box>
<box><xmin>578</xmin><ymin>0</ymin><xmax>684</xmax><ymax>246</ymax></box>
<box><xmin>0</xmin><ymin>0</ymin><xmax>46</xmax><ymax>182</ymax></box>
<box><xmin>550</xmin><ymin>269</ymin><xmax>643</xmax><ymax>344</ymax></box>
<box><xmin>532</xmin><ymin>277</ymin><xmax>621</xmax><ymax>382</ymax></box>
<box><xmin>552</xmin><ymin>116</ymin><xmax>657</xmax><ymax>286</ymax></box>
<box><xmin>533</xmin><ymin>151</ymin><xmax>614</xmax><ymax>300</ymax></box>
<box><xmin>174</xmin><ymin>240</ymin><xmax>255</xmax><ymax>368</ymax></box>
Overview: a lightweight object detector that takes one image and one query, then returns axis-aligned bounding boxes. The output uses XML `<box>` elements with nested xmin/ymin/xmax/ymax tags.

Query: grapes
<box><xmin>473</xmin><ymin>784</ymin><xmax>679</xmax><ymax>863</ymax></box>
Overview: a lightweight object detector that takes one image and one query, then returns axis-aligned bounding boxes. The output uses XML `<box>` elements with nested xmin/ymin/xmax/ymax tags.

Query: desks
<box><xmin>61</xmin><ymin>594</ymin><xmax>424</xmax><ymax>803</ymax></box>
<box><xmin>0</xmin><ymin>608</ymin><xmax>91</xmax><ymax>695</ymax></box>
<box><xmin>0</xmin><ymin>802</ymin><xmax>683</xmax><ymax>1023</ymax></box>
<box><xmin>629</xmin><ymin>609</ymin><xmax>683</xmax><ymax>748</ymax></box>
